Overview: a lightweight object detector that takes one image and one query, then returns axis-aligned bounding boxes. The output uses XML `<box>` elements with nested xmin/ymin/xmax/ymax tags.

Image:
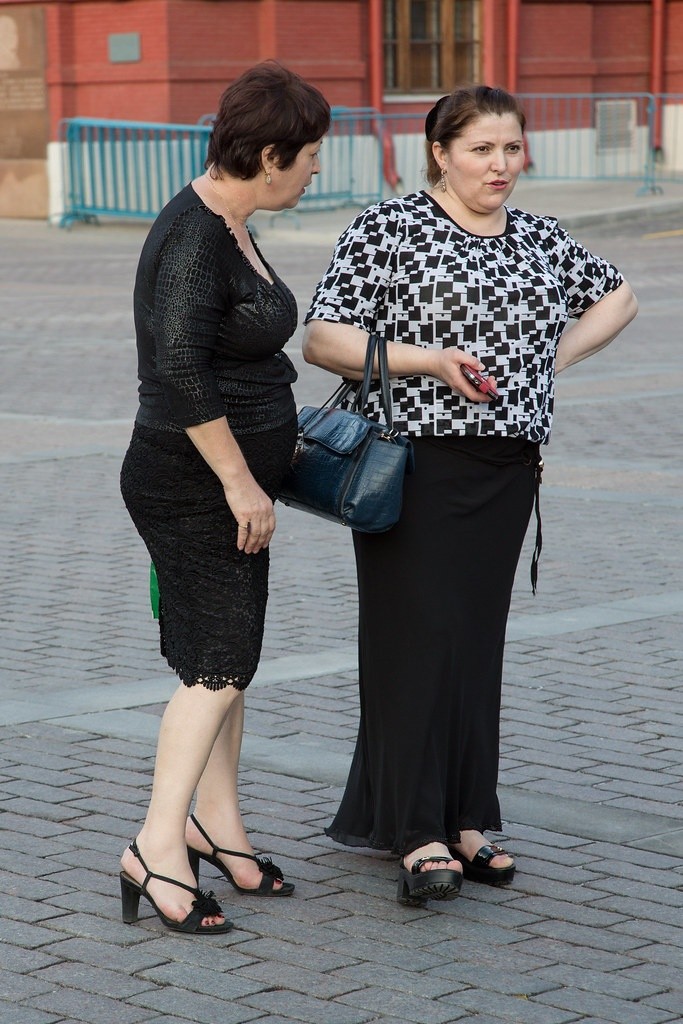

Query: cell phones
<box><xmin>461</xmin><ymin>364</ymin><xmax>499</xmax><ymax>401</ymax></box>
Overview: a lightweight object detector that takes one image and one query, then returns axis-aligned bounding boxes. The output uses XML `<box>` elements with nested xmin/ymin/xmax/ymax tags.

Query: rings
<box><xmin>239</xmin><ymin>525</ymin><xmax>249</xmax><ymax>529</ymax></box>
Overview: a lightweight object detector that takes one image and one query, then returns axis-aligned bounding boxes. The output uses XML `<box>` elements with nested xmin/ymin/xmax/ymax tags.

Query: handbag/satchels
<box><xmin>277</xmin><ymin>334</ymin><xmax>415</xmax><ymax>534</ymax></box>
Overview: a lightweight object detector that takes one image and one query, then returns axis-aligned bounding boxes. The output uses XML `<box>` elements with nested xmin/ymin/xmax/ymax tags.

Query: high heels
<box><xmin>396</xmin><ymin>851</ymin><xmax>464</xmax><ymax>908</ymax></box>
<box><xmin>186</xmin><ymin>812</ymin><xmax>295</xmax><ymax>897</ymax></box>
<box><xmin>120</xmin><ymin>838</ymin><xmax>234</xmax><ymax>934</ymax></box>
<box><xmin>450</xmin><ymin>844</ymin><xmax>516</xmax><ymax>886</ymax></box>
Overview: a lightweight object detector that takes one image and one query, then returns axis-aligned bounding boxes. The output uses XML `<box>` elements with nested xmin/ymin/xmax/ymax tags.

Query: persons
<box><xmin>117</xmin><ymin>59</ymin><xmax>333</xmax><ymax>933</ymax></box>
<box><xmin>301</xmin><ymin>87</ymin><xmax>640</xmax><ymax>909</ymax></box>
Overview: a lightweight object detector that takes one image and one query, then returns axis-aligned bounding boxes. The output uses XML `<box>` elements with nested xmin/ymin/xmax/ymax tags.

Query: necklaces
<box><xmin>203</xmin><ymin>174</ymin><xmax>237</xmax><ymax>225</ymax></box>
